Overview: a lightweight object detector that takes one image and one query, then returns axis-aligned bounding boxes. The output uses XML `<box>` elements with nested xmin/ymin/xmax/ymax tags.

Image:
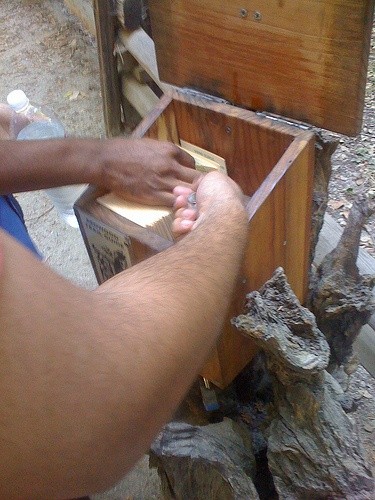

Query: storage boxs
<box><xmin>74</xmin><ymin>0</ymin><xmax>375</xmax><ymax>389</ymax></box>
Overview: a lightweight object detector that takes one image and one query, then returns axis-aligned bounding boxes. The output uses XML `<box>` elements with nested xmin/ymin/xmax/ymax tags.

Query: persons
<box><xmin>1</xmin><ymin>170</ymin><xmax>248</xmax><ymax>500</ymax></box>
<box><xmin>0</xmin><ymin>103</ymin><xmax>200</xmax><ymax>261</ymax></box>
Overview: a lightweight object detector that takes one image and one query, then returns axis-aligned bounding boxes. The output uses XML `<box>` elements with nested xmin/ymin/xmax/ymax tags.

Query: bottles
<box><xmin>7</xmin><ymin>89</ymin><xmax>90</xmax><ymax>228</ymax></box>
